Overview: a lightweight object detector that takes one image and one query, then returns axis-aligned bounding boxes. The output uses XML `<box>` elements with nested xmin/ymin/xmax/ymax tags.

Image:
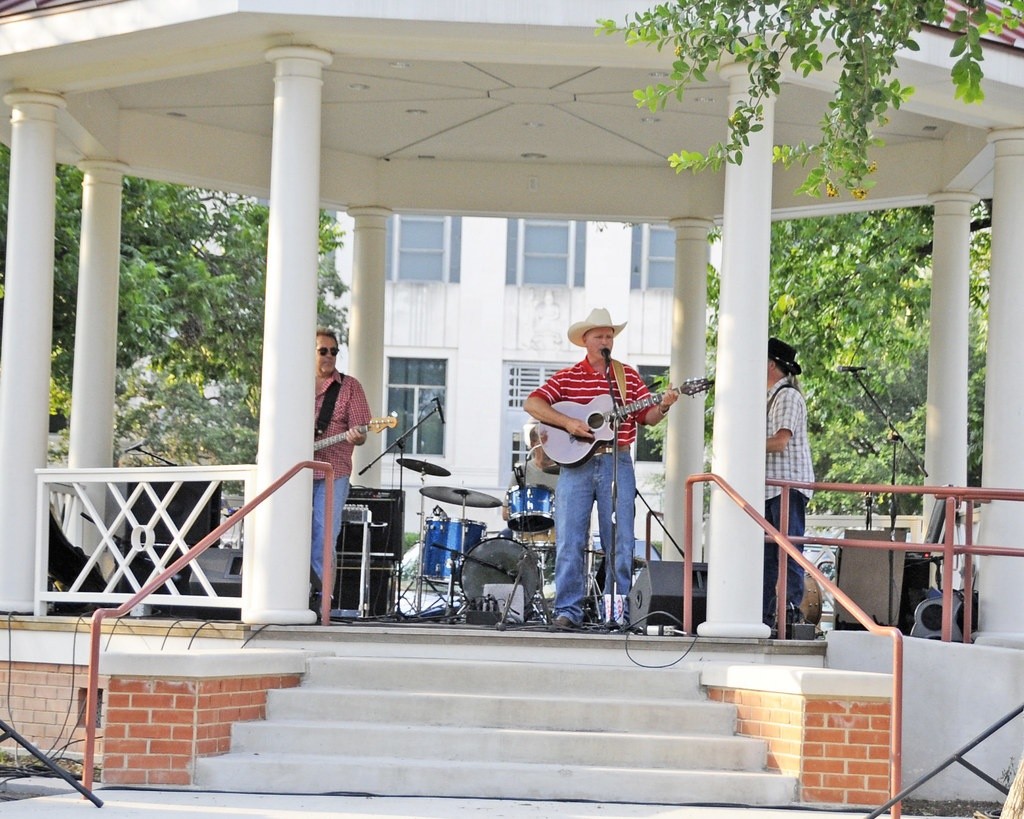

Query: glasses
<box><xmin>316</xmin><ymin>345</ymin><xmax>340</xmax><ymax>358</ymax></box>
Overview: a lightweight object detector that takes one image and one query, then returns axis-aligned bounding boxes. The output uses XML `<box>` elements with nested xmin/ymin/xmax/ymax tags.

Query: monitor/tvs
<box><xmin>923</xmin><ymin>484</ymin><xmax>953</xmax><ymax>543</ymax></box>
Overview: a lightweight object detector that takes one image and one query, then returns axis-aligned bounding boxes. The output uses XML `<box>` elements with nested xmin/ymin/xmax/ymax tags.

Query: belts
<box><xmin>595</xmin><ymin>444</ymin><xmax>630</xmax><ymax>454</ymax></box>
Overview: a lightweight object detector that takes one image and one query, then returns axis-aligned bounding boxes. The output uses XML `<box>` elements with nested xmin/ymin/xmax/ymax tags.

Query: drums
<box><xmin>456</xmin><ymin>535</ymin><xmax>541</xmax><ymax>615</ymax></box>
<box><xmin>507</xmin><ymin>483</ymin><xmax>556</xmax><ymax>535</ymax></box>
<box><xmin>420</xmin><ymin>516</ymin><xmax>488</xmax><ymax>584</ymax></box>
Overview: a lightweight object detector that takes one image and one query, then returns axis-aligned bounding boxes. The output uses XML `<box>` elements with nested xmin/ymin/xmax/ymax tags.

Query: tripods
<box><xmin>392</xmin><ymin>470</ymin><xmax>460</xmax><ymax>623</ymax></box>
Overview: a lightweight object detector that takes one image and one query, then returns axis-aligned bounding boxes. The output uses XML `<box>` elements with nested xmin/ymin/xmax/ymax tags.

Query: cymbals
<box><xmin>418</xmin><ymin>485</ymin><xmax>503</xmax><ymax>509</ymax></box>
<box><xmin>397</xmin><ymin>457</ymin><xmax>452</xmax><ymax>478</ymax></box>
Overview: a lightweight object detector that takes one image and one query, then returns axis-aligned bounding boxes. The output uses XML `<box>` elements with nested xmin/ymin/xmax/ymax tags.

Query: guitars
<box><xmin>538</xmin><ymin>378</ymin><xmax>715</xmax><ymax>470</ymax></box>
<box><xmin>312</xmin><ymin>414</ymin><xmax>397</xmax><ymax>453</ymax></box>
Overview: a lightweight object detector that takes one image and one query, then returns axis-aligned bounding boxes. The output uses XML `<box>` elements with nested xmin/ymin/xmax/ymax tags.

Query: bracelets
<box><xmin>658</xmin><ymin>405</ymin><xmax>671</xmax><ymax>415</ymax></box>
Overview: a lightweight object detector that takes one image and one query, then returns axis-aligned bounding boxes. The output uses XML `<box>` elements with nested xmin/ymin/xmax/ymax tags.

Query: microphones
<box><xmin>436</xmin><ymin>396</ymin><xmax>445</xmax><ymax>424</ymax></box>
<box><xmin>508</xmin><ymin>569</ymin><xmax>519</xmax><ymax>579</ymax></box>
<box><xmin>125</xmin><ymin>440</ymin><xmax>149</xmax><ymax>452</ymax></box>
<box><xmin>837</xmin><ymin>366</ymin><xmax>866</xmax><ymax>372</ymax></box>
<box><xmin>601</xmin><ymin>347</ymin><xmax>611</xmax><ymax>366</ymax></box>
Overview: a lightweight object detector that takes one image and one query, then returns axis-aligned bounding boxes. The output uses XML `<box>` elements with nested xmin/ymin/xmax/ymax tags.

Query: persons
<box><xmin>523</xmin><ymin>308</ymin><xmax>679</xmax><ymax>628</ymax></box>
<box><xmin>310</xmin><ymin>329</ymin><xmax>372</xmax><ymax>594</ymax></box>
<box><xmin>764</xmin><ymin>338</ymin><xmax>815</xmax><ymax>637</ymax></box>
<box><xmin>498</xmin><ymin>418</ymin><xmax>561</xmax><ymax>539</ymax></box>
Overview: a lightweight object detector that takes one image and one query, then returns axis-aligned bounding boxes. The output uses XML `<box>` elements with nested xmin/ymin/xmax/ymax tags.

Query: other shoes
<box><xmin>549</xmin><ymin>615</ymin><xmax>575</xmax><ymax>628</ymax></box>
<box><xmin>763</xmin><ymin>614</ymin><xmax>792</xmax><ymax>637</ymax></box>
<box><xmin>609</xmin><ymin>621</ymin><xmax>642</xmax><ymax>632</ymax></box>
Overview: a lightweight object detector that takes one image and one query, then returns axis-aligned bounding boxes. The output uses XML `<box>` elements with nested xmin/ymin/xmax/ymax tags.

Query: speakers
<box><xmin>189</xmin><ymin>548</ymin><xmax>243</xmax><ymax>620</ymax></box>
<box><xmin>628</xmin><ymin>560</ymin><xmax>707</xmax><ymax>636</ymax></box>
<box><xmin>330</xmin><ymin>488</ymin><xmax>406</xmax><ymax>616</ymax></box>
<box><xmin>118</xmin><ymin>481</ymin><xmax>223</xmax><ymax>614</ymax></box>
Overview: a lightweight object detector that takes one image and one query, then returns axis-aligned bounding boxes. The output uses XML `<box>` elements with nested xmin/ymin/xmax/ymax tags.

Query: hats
<box><xmin>768</xmin><ymin>338</ymin><xmax>802</xmax><ymax>375</ymax></box>
<box><xmin>567</xmin><ymin>307</ymin><xmax>628</xmax><ymax>347</ymax></box>
